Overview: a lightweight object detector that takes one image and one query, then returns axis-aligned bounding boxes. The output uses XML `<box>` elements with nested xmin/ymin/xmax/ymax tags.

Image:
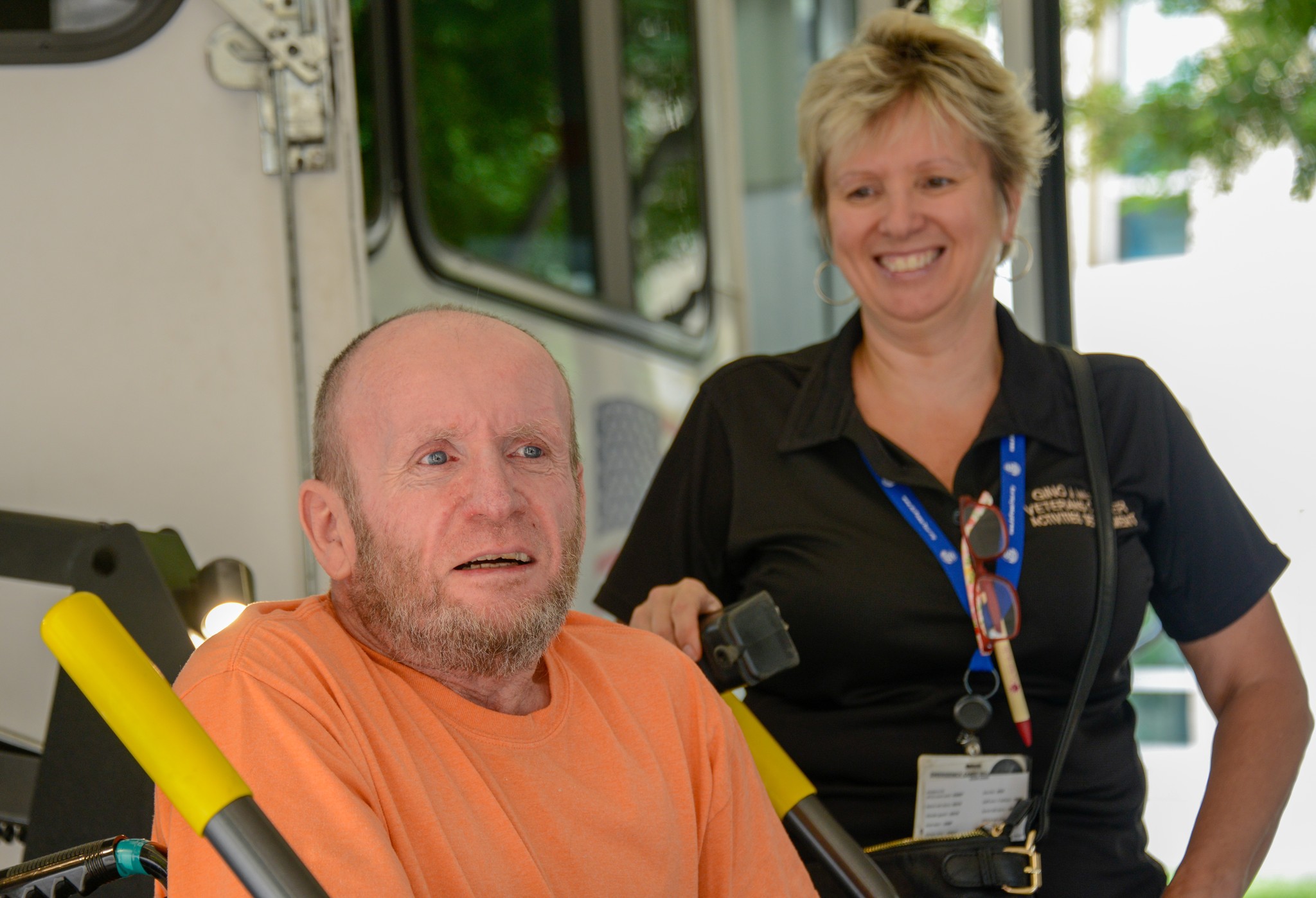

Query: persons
<box><xmin>594</xmin><ymin>9</ymin><xmax>1315</xmax><ymax>897</ymax></box>
<box><xmin>153</xmin><ymin>303</ymin><xmax>821</xmax><ymax>898</ymax></box>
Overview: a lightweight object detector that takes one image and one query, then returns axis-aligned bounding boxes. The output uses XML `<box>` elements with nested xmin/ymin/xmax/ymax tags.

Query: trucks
<box><xmin>1</xmin><ymin>1</ymin><xmax>824</xmax><ymax>898</ymax></box>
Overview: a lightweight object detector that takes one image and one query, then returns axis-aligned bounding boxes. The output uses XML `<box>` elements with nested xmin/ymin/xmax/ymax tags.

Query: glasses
<box><xmin>959</xmin><ymin>495</ymin><xmax>1020</xmax><ymax>654</ymax></box>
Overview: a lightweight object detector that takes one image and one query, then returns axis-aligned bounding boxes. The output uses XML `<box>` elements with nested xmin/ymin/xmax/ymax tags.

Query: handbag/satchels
<box><xmin>848</xmin><ymin>797</ymin><xmax>1043</xmax><ymax>898</ymax></box>
